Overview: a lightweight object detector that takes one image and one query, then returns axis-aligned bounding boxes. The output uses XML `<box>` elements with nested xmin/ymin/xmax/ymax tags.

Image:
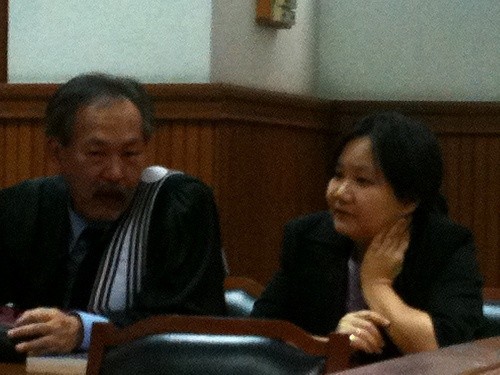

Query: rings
<box><xmin>355</xmin><ymin>328</ymin><xmax>361</xmax><ymax>337</ymax></box>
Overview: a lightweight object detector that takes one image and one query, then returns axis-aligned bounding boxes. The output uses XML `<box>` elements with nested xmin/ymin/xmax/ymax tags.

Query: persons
<box><xmin>249</xmin><ymin>103</ymin><xmax>484</xmax><ymax>368</ymax></box>
<box><xmin>0</xmin><ymin>72</ymin><xmax>226</xmax><ymax>361</ymax></box>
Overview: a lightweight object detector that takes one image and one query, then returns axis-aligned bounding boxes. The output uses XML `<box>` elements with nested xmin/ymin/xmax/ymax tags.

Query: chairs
<box><xmin>85</xmin><ymin>277</ymin><xmax>500</xmax><ymax>375</ymax></box>
